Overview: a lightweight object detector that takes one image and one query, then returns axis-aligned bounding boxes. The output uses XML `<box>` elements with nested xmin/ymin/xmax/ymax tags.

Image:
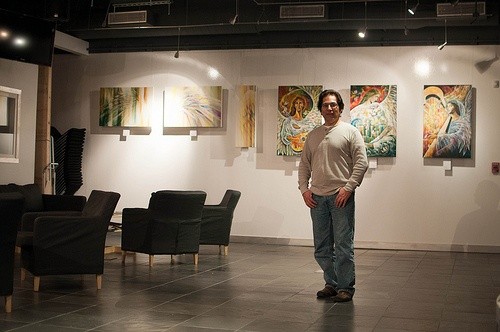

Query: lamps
<box><xmin>407</xmin><ymin>1</ymin><xmax>421</xmax><ymax>15</ymax></box>
<box><xmin>358</xmin><ymin>0</ymin><xmax>369</xmax><ymax>38</ymax></box>
<box><xmin>436</xmin><ymin>1</ymin><xmax>480</xmax><ymax>51</ymax></box>
<box><xmin>174</xmin><ymin>27</ymin><xmax>181</xmax><ymax>59</ymax></box>
<box><xmin>229</xmin><ymin>1</ymin><xmax>241</xmax><ymax>23</ymax></box>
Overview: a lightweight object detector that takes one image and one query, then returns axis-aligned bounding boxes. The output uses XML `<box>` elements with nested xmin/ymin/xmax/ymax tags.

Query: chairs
<box><xmin>0</xmin><ymin>127</ymin><xmax>242</xmax><ymax>313</ymax></box>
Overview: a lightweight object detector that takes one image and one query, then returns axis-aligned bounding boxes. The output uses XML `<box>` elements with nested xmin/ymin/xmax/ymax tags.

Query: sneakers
<box><xmin>333</xmin><ymin>291</ymin><xmax>352</xmax><ymax>301</ymax></box>
<box><xmin>317</xmin><ymin>287</ymin><xmax>337</xmax><ymax>298</ymax></box>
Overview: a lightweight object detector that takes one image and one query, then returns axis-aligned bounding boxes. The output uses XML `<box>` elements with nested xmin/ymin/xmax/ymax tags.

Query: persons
<box><xmin>298</xmin><ymin>90</ymin><xmax>370</xmax><ymax>302</ymax></box>
